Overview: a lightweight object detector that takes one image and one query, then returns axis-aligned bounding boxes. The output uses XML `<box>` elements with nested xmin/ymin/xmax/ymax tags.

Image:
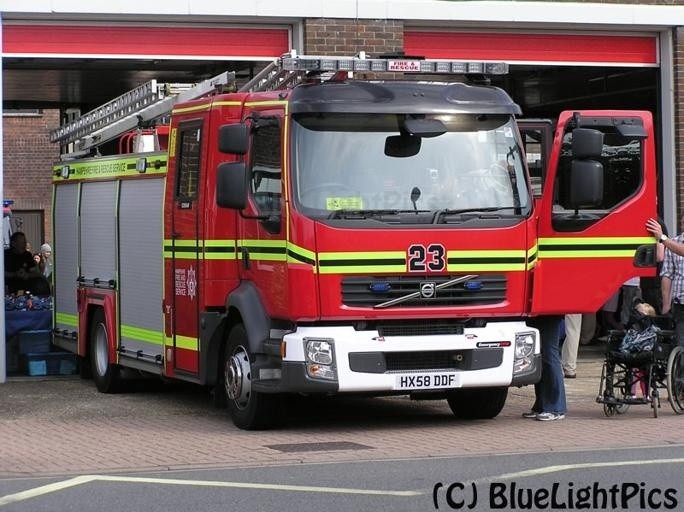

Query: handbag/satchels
<box><xmin>619</xmin><ymin>315</ymin><xmax>661</xmax><ymax>353</ymax></box>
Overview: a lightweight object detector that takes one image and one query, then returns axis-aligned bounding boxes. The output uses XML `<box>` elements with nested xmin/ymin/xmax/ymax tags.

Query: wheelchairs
<box><xmin>594</xmin><ymin>295</ymin><xmax>684</xmax><ymax>420</ymax></box>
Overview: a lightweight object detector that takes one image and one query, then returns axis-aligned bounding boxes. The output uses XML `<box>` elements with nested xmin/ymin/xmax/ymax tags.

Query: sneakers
<box><xmin>535</xmin><ymin>410</ymin><xmax>565</xmax><ymax>421</ymax></box>
<box><xmin>522</xmin><ymin>410</ymin><xmax>543</xmax><ymax>418</ymax></box>
<box><xmin>579</xmin><ymin>337</ymin><xmax>606</xmax><ymax>346</ymax></box>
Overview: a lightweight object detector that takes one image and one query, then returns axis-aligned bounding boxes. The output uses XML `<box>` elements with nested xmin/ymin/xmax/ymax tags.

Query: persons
<box><xmin>561</xmin><ymin>312</ymin><xmax>583</xmax><ymax>378</ymax></box>
<box><xmin>524</xmin><ymin>310</ymin><xmax>566</xmax><ymax>422</ymax></box>
<box><xmin>4</xmin><ymin>230</ymin><xmax>52</xmax><ymax>296</ymax></box>
<box><xmin>646</xmin><ymin>216</ymin><xmax>684</xmax><ymax>258</ymax></box>
<box><xmin>661</xmin><ymin>214</ymin><xmax>684</xmax><ymax>348</ymax></box>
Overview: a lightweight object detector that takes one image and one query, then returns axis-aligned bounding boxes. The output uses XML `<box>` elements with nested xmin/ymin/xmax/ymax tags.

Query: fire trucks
<box><xmin>47</xmin><ymin>46</ymin><xmax>661</xmax><ymax>433</ymax></box>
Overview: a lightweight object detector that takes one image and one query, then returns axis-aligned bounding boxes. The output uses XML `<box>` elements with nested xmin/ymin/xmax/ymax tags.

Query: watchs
<box><xmin>658</xmin><ymin>233</ymin><xmax>669</xmax><ymax>243</ymax></box>
